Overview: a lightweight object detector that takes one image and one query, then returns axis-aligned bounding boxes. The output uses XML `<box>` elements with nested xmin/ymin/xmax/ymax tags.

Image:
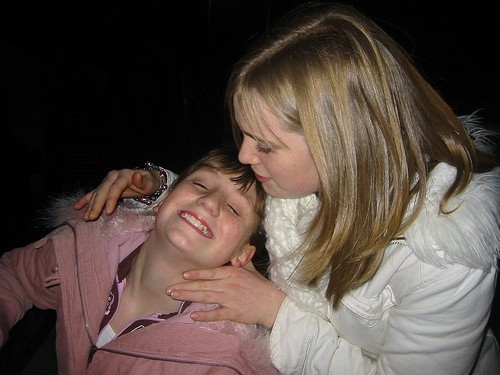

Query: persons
<box><xmin>0</xmin><ymin>141</ymin><xmax>286</xmax><ymax>375</ymax></box>
<box><xmin>72</xmin><ymin>3</ymin><xmax>500</xmax><ymax>375</ymax></box>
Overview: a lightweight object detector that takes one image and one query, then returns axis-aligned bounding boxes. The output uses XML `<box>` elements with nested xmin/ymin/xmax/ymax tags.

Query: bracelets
<box><xmin>131</xmin><ymin>160</ymin><xmax>168</xmax><ymax>206</ymax></box>
